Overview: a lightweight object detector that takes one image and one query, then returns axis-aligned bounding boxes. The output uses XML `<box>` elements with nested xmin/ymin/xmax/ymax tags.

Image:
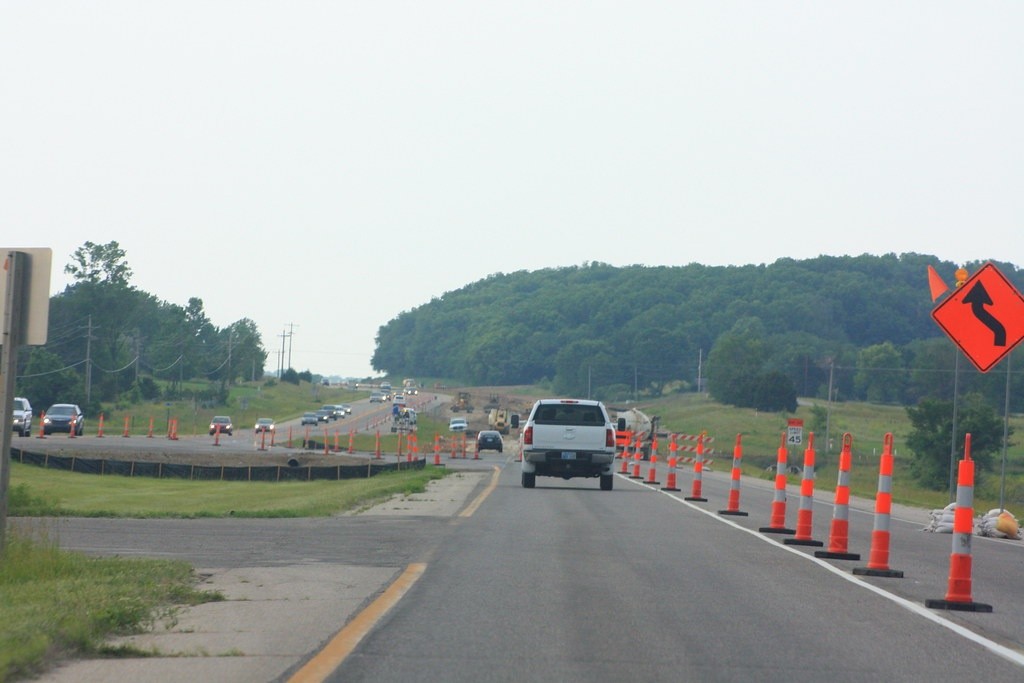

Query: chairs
<box><xmin>542</xmin><ymin>410</ymin><xmax>556</xmax><ymax>420</ymax></box>
<box><xmin>585</xmin><ymin>413</ymin><xmax>597</xmax><ymax>422</ymax></box>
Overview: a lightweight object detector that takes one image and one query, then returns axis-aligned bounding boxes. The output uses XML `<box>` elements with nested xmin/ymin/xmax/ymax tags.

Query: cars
<box><xmin>43</xmin><ymin>404</ymin><xmax>84</xmax><ymax>436</ymax></box>
<box><xmin>208</xmin><ymin>416</ymin><xmax>233</xmax><ymax>436</ymax></box>
<box><xmin>447</xmin><ymin>417</ymin><xmax>469</xmax><ymax>433</ymax></box>
<box><xmin>369</xmin><ymin>379</ymin><xmax>418</xmax><ymax>433</ymax></box>
<box><xmin>477</xmin><ymin>431</ymin><xmax>504</xmax><ymax>453</ymax></box>
<box><xmin>254</xmin><ymin>418</ymin><xmax>276</xmax><ymax>434</ymax></box>
<box><xmin>301</xmin><ymin>404</ymin><xmax>352</xmax><ymax>426</ymax></box>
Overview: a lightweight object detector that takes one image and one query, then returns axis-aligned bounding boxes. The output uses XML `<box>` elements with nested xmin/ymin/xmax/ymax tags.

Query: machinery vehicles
<box><xmin>450</xmin><ymin>391</ymin><xmax>519</xmax><ymax>435</ymax></box>
<box><xmin>614</xmin><ymin>408</ymin><xmax>655</xmax><ymax>460</ymax></box>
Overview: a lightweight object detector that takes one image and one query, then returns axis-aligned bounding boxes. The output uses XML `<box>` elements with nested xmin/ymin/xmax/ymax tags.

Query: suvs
<box><xmin>12</xmin><ymin>397</ymin><xmax>32</xmax><ymax>437</ymax></box>
<box><xmin>521</xmin><ymin>399</ymin><xmax>615</xmax><ymax>491</ymax></box>
